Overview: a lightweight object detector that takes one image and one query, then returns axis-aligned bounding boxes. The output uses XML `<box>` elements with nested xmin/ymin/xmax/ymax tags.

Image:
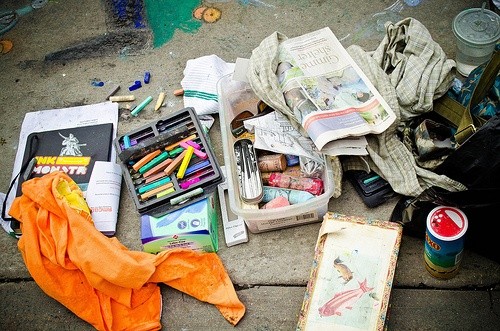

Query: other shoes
<box><xmin>31</xmin><ymin>0</ymin><xmax>48</xmax><ymax>8</ymax></box>
<box><xmin>1</xmin><ymin>40</ymin><xmax>13</xmax><ymax>54</ymax></box>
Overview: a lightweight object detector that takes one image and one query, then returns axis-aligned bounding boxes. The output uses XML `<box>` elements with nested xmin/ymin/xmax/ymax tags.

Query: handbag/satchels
<box><xmin>390</xmin><ymin>114</ymin><xmax>500</xmax><ymax>260</ymax></box>
<box><xmin>459</xmin><ymin>43</ymin><xmax>499</xmax><ymax>119</ymax></box>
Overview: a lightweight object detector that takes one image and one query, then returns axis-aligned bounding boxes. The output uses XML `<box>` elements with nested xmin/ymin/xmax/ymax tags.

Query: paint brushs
<box><xmin>239</xmin><ymin>142</ymin><xmax>259</xmax><ymax>199</ymax></box>
<box><xmin>92</xmin><ymin>72</ymin><xmax>184</xmax><ymax>120</ymax></box>
<box><xmin>120</xmin><ymin>127</ymin><xmax>211</xmax><ymax>206</ymax></box>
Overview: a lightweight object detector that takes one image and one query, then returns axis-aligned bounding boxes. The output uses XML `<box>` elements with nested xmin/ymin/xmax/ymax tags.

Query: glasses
<box><xmin>230</xmin><ymin>100</ymin><xmax>275</xmax><ymax>138</ymax></box>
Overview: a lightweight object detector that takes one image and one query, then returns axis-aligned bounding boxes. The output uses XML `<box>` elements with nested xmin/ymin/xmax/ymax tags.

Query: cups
<box><xmin>452</xmin><ymin>8</ymin><xmax>500</xmax><ymax>77</ymax></box>
